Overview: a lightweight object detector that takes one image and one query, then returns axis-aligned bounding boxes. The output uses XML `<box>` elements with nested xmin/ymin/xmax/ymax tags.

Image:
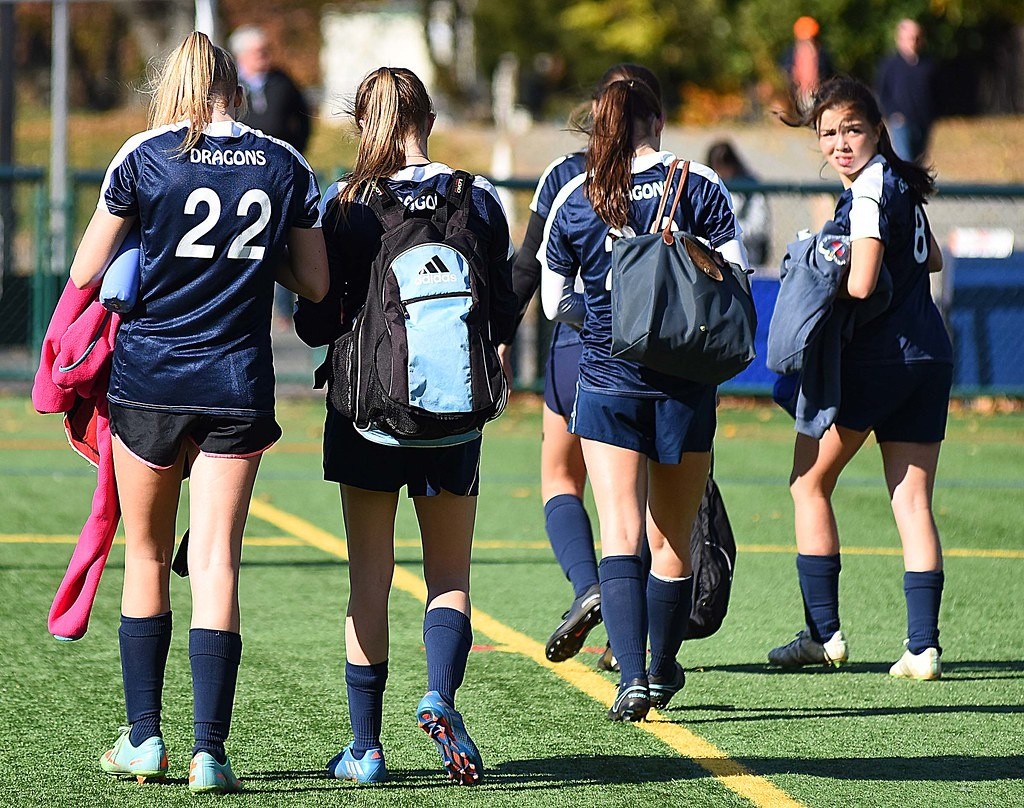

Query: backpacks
<box><xmin>319</xmin><ymin>171</ymin><xmax>508</xmax><ymax>439</ymax></box>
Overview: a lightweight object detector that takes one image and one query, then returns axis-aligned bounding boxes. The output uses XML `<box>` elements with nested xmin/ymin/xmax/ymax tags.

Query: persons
<box><xmin>768</xmin><ymin>78</ymin><xmax>954</xmax><ymax>678</ymax></box>
<box><xmin>709</xmin><ymin>142</ymin><xmax>770</xmax><ymax>269</ymax></box>
<box><xmin>293</xmin><ymin>68</ymin><xmax>519</xmax><ymax>782</ymax></box>
<box><xmin>780</xmin><ymin>37</ymin><xmax>829</xmax><ymax>122</ymax></box>
<box><xmin>871</xmin><ymin>17</ymin><xmax>947</xmax><ymax>167</ymax></box>
<box><xmin>230</xmin><ymin>26</ymin><xmax>310</xmax><ymax>330</ymax></box>
<box><xmin>67</xmin><ymin>30</ymin><xmax>330</xmax><ymax>794</ymax></box>
<box><xmin>479</xmin><ymin>61</ymin><xmax>752</xmax><ymax>722</ymax></box>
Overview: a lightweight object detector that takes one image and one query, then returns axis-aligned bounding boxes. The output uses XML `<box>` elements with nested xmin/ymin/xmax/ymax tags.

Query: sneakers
<box><xmin>890</xmin><ymin>640</ymin><xmax>941</xmax><ymax>680</ymax></box>
<box><xmin>415</xmin><ymin>692</ymin><xmax>484</xmax><ymax>784</ymax></box>
<box><xmin>767</xmin><ymin>620</ymin><xmax>848</xmax><ymax>670</ymax></box>
<box><xmin>607</xmin><ymin>677</ymin><xmax>651</xmax><ymax>721</ymax></box>
<box><xmin>324</xmin><ymin>741</ymin><xmax>386</xmax><ymax>783</ymax></box>
<box><xmin>99</xmin><ymin>726</ymin><xmax>169</xmax><ymax>784</ymax></box>
<box><xmin>597</xmin><ymin>639</ymin><xmax>623</xmax><ymax>671</ymax></box>
<box><xmin>646</xmin><ymin>662</ymin><xmax>685</xmax><ymax>707</ymax></box>
<box><xmin>544</xmin><ymin>584</ymin><xmax>608</xmax><ymax>662</ymax></box>
<box><xmin>187</xmin><ymin>753</ymin><xmax>242</xmax><ymax>794</ymax></box>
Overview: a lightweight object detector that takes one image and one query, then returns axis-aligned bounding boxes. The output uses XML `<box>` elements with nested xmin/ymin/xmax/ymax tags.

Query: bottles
<box><xmin>795</xmin><ymin>227</ymin><xmax>812</xmax><ymax>242</ymax></box>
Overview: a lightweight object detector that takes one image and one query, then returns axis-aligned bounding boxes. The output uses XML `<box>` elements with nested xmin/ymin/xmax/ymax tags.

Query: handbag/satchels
<box><xmin>683</xmin><ymin>452</ymin><xmax>738</xmax><ymax>640</ymax></box>
<box><xmin>610</xmin><ymin>162</ymin><xmax>757</xmax><ymax>385</ymax></box>
